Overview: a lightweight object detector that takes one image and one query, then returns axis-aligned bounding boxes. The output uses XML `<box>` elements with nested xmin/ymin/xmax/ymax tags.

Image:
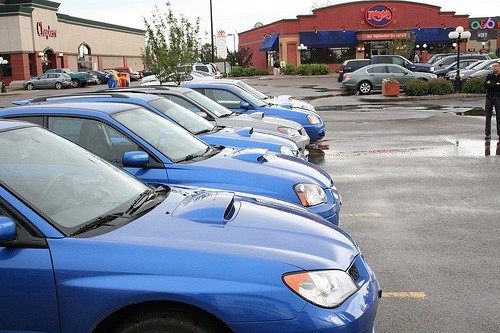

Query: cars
<box><xmin>0</xmin><ymin>101</ymin><xmax>342</xmax><ymax>228</ymax></box>
<box><xmin>23</xmin><ymin>73</ymin><xmax>71</xmax><ymax>90</ymax></box>
<box><xmin>141</xmin><ymin>61</ymin><xmax>326</xmax><ymax>143</ymax></box>
<box><xmin>0</xmin><ymin>118</ymin><xmax>382</xmax><ymax>333</ymax></box>
<box><xmin>342</xmin><ymin>63</ymin><xmax>438</xmax><ymax>95</ymax></box>
<box><xmin>424</xmin><ymin>52</ymin><xmax>500</xmax><ymax>83</ymax></box>
<box><xmin>12</xmin><ymin>91</ymin><xmax>309</xmax><ymax>162</ymax></box>
<box><xmin>95</xmin><ymin>85</ymin><xmax>310</xmax><ymax>157</ymax></box>
<box><xmin>87</xmin><ymin>66</ymin><xmax>153</xmax><ymax>85</ymax></box>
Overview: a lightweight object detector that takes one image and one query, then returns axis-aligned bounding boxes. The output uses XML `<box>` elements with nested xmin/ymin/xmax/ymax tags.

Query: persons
<box><xmin>483</xmin><ymin>62</ymin><xmax>500</xmax><ymax>139</ymax></box>
<box><xmin>415</xmin><ymin>52</ymin><xmax>419</xmax><ymax>63</ymax></box>
<box><xmin>274</xmin><ymin>58</ymin><xmax>286</xmax><ymax>75</ymax></box>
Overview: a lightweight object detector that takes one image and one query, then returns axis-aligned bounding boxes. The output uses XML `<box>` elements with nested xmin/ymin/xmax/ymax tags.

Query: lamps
<box><xmin>441</xmin><ymin>22</ymin><xmax>447</xmax><ymax>30</ymax></box>
<box><xmin>264</xmin><ymin>30</ymin><xmax>275</xmax><ymax>38</ymax></box>
<box><xmin>416</xmin><ymin>23</ymin><xmax>421</xmax><ymax>31</ymax></box>
<box><xmin>313</xmin><ymin>26</ymin><xmax>317</xmax><ymax>33</ymax></box>
<box><xmin>57</xmin><ymin>53</ymin><xmax>63</xmax><ymax>58</ymax></box>
<box><xmin>38</xmin><ymin>52</ymin><xmax>44</xmax><ymax>57</ymax></box>
<box><xmin>341</xmin><ymin>25</ymin><xmax>345</xmax><ymax>33</ymax></box>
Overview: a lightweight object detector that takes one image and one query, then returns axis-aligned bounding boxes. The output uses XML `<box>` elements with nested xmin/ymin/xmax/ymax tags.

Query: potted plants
<box><xmin>381</xmin><ymin>72</ymin><xmax>400</xmax><ymax>96</ymax></box>
<box><xmin>273</xmin><ymin>62</ymin><xmax>281</xmax><ymax>75</ymax></box>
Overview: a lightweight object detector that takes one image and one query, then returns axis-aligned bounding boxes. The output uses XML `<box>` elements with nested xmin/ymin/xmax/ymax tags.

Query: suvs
<box><xmin>45</xmin><ymin>68</ymin><xmax>87</xmax><ymax>88</ymax></box>
<box><xmin>342</xmin><ymin>58</ymin><xmax>370</xmax><ymax>71</ymax></box>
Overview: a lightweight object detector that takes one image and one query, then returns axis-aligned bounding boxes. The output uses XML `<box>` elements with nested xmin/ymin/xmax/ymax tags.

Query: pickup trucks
<box><xmin>337</xmin><ymin>55</ymin><xmax>435</xmax><ymax>82</ymax></box>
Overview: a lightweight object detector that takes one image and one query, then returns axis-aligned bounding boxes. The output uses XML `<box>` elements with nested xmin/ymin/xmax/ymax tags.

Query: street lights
<box><xmin>448</xmin><ymin>26</ymin><xmax>471</xmax><ymax>91</ymax></box>
<box><xmin>297</xmin><ymin>43</ymin><xmax>308</xmax><ymax>65</ymax></box>
<box><xmin>0</xmin><ymin>57</ymin><xmax>9</xmax><ymax>93</ymax></box>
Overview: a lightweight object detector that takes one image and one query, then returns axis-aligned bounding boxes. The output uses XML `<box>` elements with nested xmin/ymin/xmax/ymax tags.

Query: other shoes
<box><xmin>485</xmin><ymin>135</ymin><xmax>491</xmax><ymax>139</ymax></box>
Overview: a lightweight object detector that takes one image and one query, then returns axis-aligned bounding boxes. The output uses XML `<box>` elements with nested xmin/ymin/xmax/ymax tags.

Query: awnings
<box><xmin>299</xmin><ymin>31</ymin><xmax>358</xmax><ymax>48</ymax></box>
<box><xmin>411</xmin><ymin>28</ymin><xmax>455</xmax><ymax>44</ymax></box>
<box><xmin>259</xmin><ymin>34</ymin><xmax>279</xmax><ymax>52</ymax></box>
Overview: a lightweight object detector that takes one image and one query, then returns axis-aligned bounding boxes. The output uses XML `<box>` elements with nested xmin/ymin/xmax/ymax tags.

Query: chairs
<box><xmin>77</xmin><ymin>122</ymin><xmax>105</xmax><ymax>155</ymax></box>
